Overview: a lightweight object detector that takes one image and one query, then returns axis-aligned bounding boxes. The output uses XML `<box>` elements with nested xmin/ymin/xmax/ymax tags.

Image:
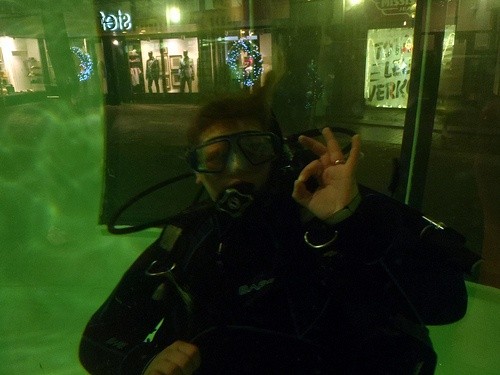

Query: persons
<box><xmin>177</xmin><ymin>49</ymin><xmax>196</xmax><ymax>96</ymax></box>
<box><xmin>145</xmin><ymin>51</ymin><xmax>161</xmax><ymax>93</ymax></box>
<box><xmin>78</xmin><ymin>93</ymin><xmax>481</xmax><ymax>375</ymax></box>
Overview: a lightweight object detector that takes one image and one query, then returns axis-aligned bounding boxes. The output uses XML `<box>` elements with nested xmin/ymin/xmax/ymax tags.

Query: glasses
<box><xmin>182</xmin><ymin>129</ymin><xmax>283</xmax><ymax>173</ymax></box>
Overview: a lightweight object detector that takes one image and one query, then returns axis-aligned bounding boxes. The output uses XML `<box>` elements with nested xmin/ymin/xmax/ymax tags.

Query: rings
<box><xmin>332</xmin><ymin>159</ymin><xmax>345</xmax><ymax>165</ymax></box>
